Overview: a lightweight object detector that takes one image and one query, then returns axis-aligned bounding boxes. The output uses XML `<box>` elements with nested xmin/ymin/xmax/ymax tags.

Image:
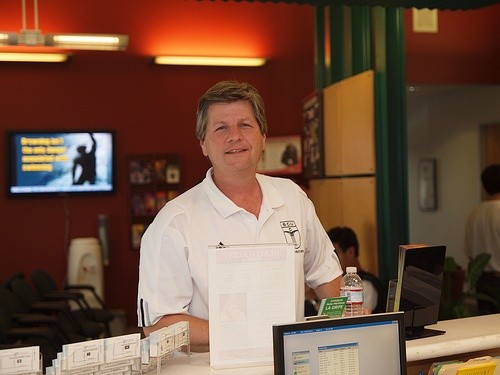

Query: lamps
<box><xmin>0</xmin><ymin>0</ymin><xmax>127</xmax><ymax>64</ymax></box>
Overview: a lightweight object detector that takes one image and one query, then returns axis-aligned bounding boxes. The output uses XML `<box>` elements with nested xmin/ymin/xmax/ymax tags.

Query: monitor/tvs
<box><xmin>4</xmin><ymin>128</ymin><xmax>117</xmax><ymax>198</ymax></box>
<box><xmin>272</xmin><ymin>311</ymin><xmax>408</xmax><ymax>375</ymax></box>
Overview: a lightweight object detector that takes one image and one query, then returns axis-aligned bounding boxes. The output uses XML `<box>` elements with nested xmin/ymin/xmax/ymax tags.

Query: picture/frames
<box><xmin>417</xmin><ymin>158</ymin><xmax>437</xmax><ymax>211</ymax></box>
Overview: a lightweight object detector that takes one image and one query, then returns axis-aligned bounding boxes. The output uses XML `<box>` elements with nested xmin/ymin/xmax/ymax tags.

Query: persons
<box><xmin>304</xmin><ymin>227</ymin><xmax>386</xmax><ymax>315</ymax></box>
<box><xmin>137</xmin><ymin>81</ymin><xmax>345</xmax><ymax>352</ymax></box>
<box><xmin>463</xmin><ymin>163</ymin><xmax>500</xmax><ymax>317</ymax></box>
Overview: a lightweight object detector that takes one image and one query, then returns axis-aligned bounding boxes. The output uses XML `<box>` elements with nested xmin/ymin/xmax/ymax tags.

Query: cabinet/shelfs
<box><xmin>128</xmin><ymin>151</ymin><xmax>182</xmax><ymax>250</ymax></box>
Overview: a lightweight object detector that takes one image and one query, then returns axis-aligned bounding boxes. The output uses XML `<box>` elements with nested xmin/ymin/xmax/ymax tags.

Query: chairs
<box><xmin>0</xmin><ymin>269</ymin><xmax>113</xmax><ymax>374</ymax></box>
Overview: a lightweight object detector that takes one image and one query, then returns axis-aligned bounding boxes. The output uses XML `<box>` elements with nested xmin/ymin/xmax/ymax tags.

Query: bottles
<box><xmin>339</xmin><ymin>266</ymin><xmax>364</xmax><ymax>317</ymax></box>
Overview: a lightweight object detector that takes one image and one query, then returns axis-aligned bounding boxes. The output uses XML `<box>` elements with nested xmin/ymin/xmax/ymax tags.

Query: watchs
<box><xmin>311</xmin><ymin>297</ymin><xmax>320</xmax><ymax>305</ymax></box>
